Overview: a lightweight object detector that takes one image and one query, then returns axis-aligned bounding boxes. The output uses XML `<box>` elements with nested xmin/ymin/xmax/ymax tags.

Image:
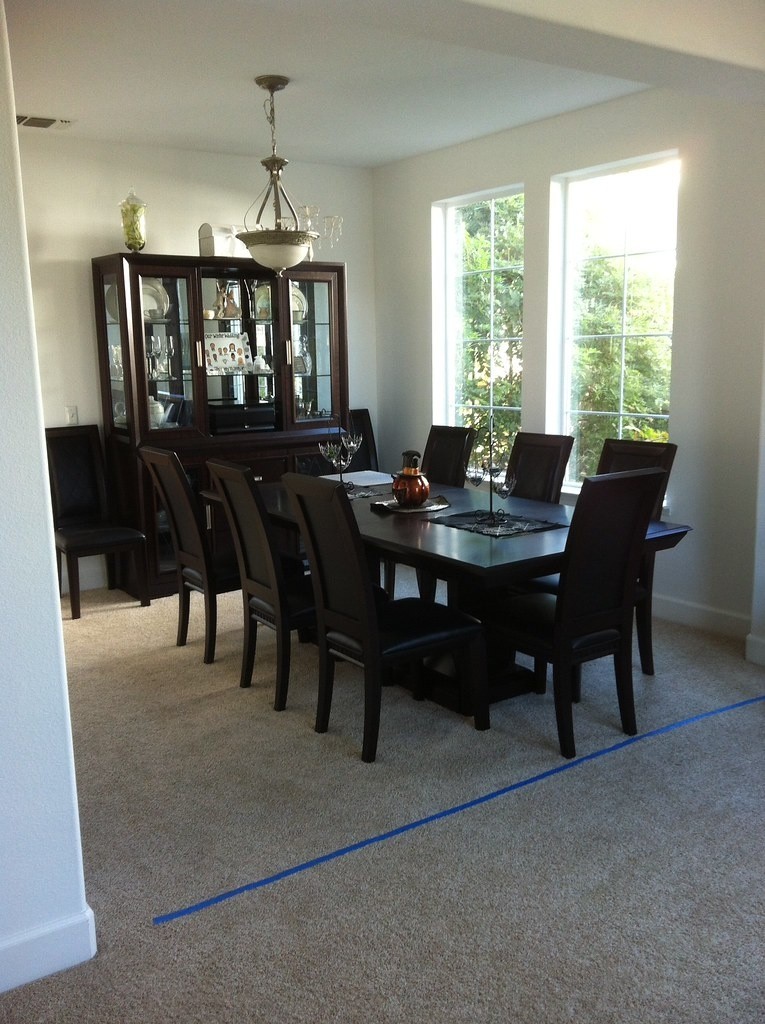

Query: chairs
<box><xmin>44</xmin><ymin>424</ymin><xmax>151</xmax><ymax>620</ymax></box>
<box><xmin>137</xmin><ymin>409</ymin><xmax>677</xmax><ymax>762</ymax></box>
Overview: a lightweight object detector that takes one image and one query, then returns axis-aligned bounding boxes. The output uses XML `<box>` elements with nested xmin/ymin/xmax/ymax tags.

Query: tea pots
<box><xmin>148</xmin><ymin>396</ymin><xmax>174</xmax><ymax>429</ymax></box>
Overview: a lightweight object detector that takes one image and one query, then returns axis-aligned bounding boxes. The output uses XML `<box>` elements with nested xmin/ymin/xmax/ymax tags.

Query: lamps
<box><xmin>235</xmin><ymin>74</ymin><xmax>321</xmax><ymax>278</ymax></box>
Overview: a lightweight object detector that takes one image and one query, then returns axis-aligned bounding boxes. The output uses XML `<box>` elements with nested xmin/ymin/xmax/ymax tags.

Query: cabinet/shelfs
<box><xmin>92</xmin><ymin>253</ymin><xmax>353</xmax><ymax>607</ymax></box>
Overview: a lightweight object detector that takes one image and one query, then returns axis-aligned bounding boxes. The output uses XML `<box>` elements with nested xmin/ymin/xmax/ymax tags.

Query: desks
<box><xmin>269</xmin><ymin>470</ymin><xmax>693</xmax><ymax>728</ymax></box>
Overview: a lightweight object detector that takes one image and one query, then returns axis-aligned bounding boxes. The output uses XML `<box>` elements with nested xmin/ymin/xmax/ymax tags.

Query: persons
<box><xmin>213</xmin><ymin>285</ymin><xmax>227</xmax><ymax>317</ymax></box>
<box><xmin>205</xmin><ymin>343</ymin><xmax>245</xmax><ymax>367</ymax></box>
